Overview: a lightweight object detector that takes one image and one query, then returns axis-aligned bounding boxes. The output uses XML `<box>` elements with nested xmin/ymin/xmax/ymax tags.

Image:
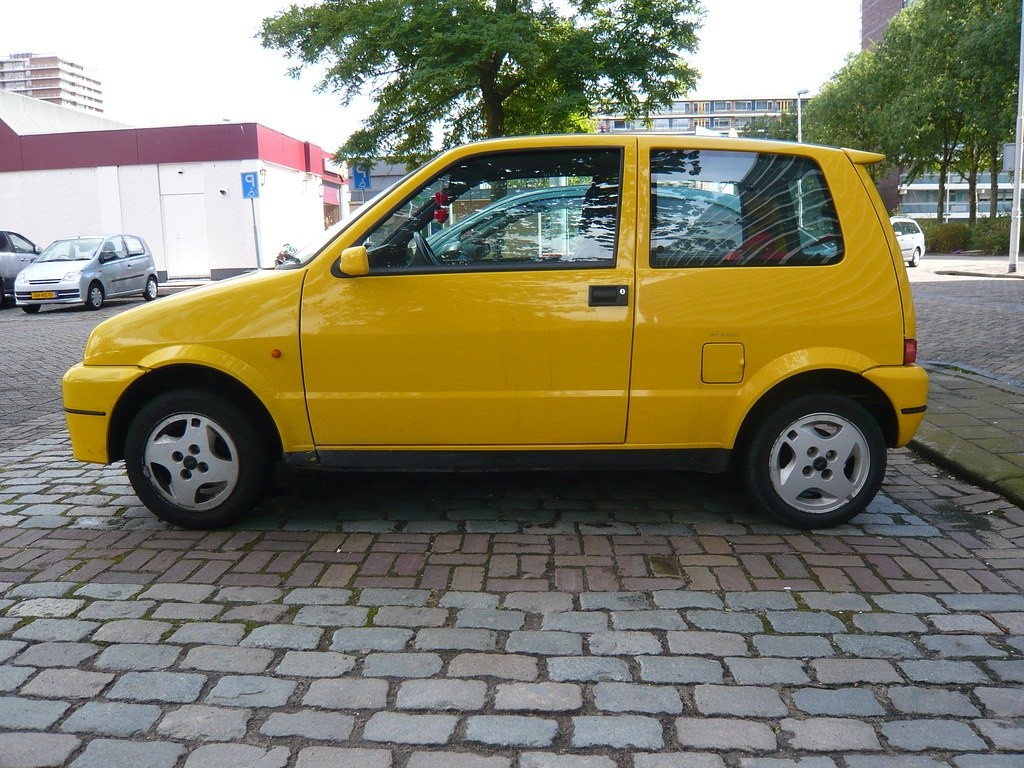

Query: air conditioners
<box><xmin>27</xmin><ymin>81</ymin><xmax>32</xmax><ymax>85</ymax></box>
<box><xmin>28</xmin><ymin>91</ymin><xmax>33</xmax><ymax>97</ymax></box>
<box><xmin>26</xmin><ymin>71</ymin><xmax>31</xmax><ymax>76</ymax></box>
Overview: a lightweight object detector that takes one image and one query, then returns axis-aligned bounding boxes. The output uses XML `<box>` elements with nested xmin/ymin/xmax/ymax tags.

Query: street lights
<box><xmin>796</xmin><ymin>87</ymin><xmax>809</xmax><ymax>228</ymax></box>
<box><xmin>943</xmin><ymin>143</ymin><xmax>965</xmax><ymax>224</ymax></box>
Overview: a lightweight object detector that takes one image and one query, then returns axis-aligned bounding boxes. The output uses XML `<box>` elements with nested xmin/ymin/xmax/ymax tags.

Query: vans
<box><xmin>0</xmin><ymin>230</ymin><xmax>50</xmax><ymax>308</ymax></box>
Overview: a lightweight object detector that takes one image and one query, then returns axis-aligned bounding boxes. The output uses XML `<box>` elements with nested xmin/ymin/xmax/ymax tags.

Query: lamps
<box><xmin>260</xmin><ymin>167</ymin><xmax>266</xmax><ymax>185</ymax></box>
<box><xmin>319</xmin><ymin>184</ymin><xmax>325</xmax><ymax>197</ymax></box>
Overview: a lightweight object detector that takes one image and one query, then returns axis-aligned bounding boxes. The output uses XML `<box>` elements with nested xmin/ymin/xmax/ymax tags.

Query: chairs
<box><xmin>97</xmin><ymin>241</ymin><xmax>115</xmax><ymax>259</ymax></box>
<box><xmin>579</xmin><ymin>178</ymin><xmax>615</xmax><ymax>262</ymax></box>
<box><xmin>78</xmin><ymin>242</ymin><xmax>91</xmax><ymax>252</ymax></box>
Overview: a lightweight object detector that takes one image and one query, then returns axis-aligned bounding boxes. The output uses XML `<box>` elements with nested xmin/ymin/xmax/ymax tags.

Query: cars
<box><xmin>404</xmin><ymin>181</ymin><xmax>833</xmax><ymax>263</ymax></box>
<box><xmin>60</xmin><ymin>134</ymin><xmax>930</xmax><ymax>532</ymax></box>
<box><xmin>889</xmin><ymin>213</ymin><xmax>926</xmax><ymax>269</ymax></box>
<box><xmin>13</xmin><ymin>234</ymin><xmax>158</xmax><ymax>315</ymax></box>
